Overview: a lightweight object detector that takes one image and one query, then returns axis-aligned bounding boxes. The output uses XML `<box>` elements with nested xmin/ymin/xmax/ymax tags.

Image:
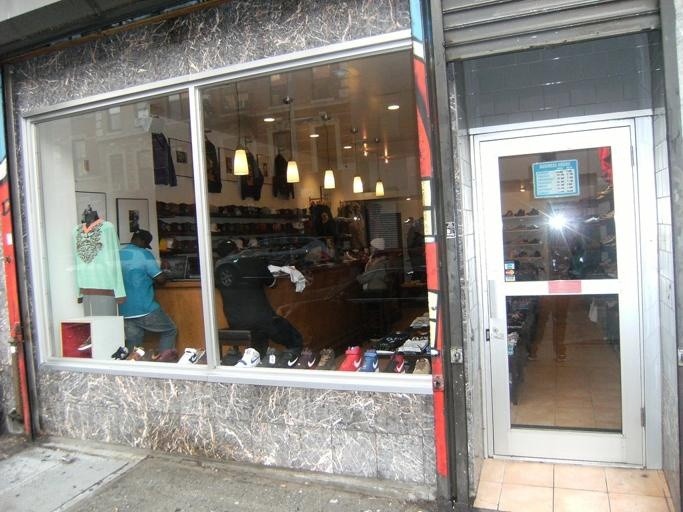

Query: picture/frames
<box><xmin>167</xmin><ymin>137</ymin><xmax>194</xmax><ymax>179</ymax></box>
<box><xmin>75</xmin><ymin>190</ymin><xmax>151</xmax><ymax>245</ymax></box>
<box><xmin>218</xmin><ymin>147</ymin><xmax>239</xmax><ymax>182</ymax></box>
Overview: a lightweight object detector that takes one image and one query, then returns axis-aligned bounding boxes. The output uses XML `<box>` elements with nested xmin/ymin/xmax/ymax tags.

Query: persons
<box><xmin>215</xmin><ymin>240</ymin><xmax>304</xmax><ymax>355</ymax></box>
<box><xmin>118</xmin><ymin>229</ymin><xmax>178</xmax><ymax>351</ymax></box>
<box><xmin>527</xmin><ymin>271</ymin><xmax>575</xmax><ymax>360</ymax></box>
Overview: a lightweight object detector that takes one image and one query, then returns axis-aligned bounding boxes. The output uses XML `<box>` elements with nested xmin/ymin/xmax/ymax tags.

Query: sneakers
<box><xmin>554</xmin><ymin>351</ymin><xmax>568</xmax><ymax>367</ymax></box>
<box><xmin>219</xmin><ymin>347</ymin><xmax>337</xmax><ymax>371</ymax></box>
<box><xmin>111</xmin><ymin>346</ymin><xmax>206</xmax><ymax>365</ymax></box>
<box><xmin>77</xmin><ymin>335</ymin><xmax>91</xmax><ymax>351</ymax></box>
<box><xmin>527</xmin><ymin>352</ymin><xmax>538</xmax><ymax>361</ymax></box>
<box><xmin>338</xmin><ymin>343</ymin><xmax>431</xmax><ymax>375</ymax></box>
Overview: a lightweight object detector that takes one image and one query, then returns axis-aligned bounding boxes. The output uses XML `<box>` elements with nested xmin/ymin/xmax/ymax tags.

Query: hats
<box><xmin>132</xmin><ymin>229</ymin><xmax>153</xmax><ymax>249</ymax></box>
<box><xmin>370</xmin><ymin>238</ymin><xmax>385</xmax><ymax>251</ymax></box>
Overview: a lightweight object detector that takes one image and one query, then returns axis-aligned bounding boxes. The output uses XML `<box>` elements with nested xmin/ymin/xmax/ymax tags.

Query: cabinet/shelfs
<box><xmin>502</xmin><ymin>214</ymin><xmax>549</xmax><ymax>280</ymax></box>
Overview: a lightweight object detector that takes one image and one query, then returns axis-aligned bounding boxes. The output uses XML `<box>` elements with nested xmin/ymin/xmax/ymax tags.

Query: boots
<box><xmin>502</xmin><ymin>207</ymin><xmax>539</xmax><ymax>217</ymax></box>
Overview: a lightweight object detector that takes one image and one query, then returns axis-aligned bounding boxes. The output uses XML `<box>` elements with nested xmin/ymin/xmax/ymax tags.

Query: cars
<box><xmin>214</xmin><ymin>232</ymin><xmax>340</xmax><ymax>293</ymax></box>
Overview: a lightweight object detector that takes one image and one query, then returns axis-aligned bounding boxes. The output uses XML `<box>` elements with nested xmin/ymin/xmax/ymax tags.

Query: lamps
<box><xmin>230</xmin><ymin>82</ymin><xmax>385</xmax><ymax>198</ymax></box>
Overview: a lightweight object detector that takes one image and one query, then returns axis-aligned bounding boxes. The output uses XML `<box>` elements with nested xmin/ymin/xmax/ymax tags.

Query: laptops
<box><xmin>159</xmin><ymin>255</ymin><xmax>188</xmax><ymax>279</ymax></box>
